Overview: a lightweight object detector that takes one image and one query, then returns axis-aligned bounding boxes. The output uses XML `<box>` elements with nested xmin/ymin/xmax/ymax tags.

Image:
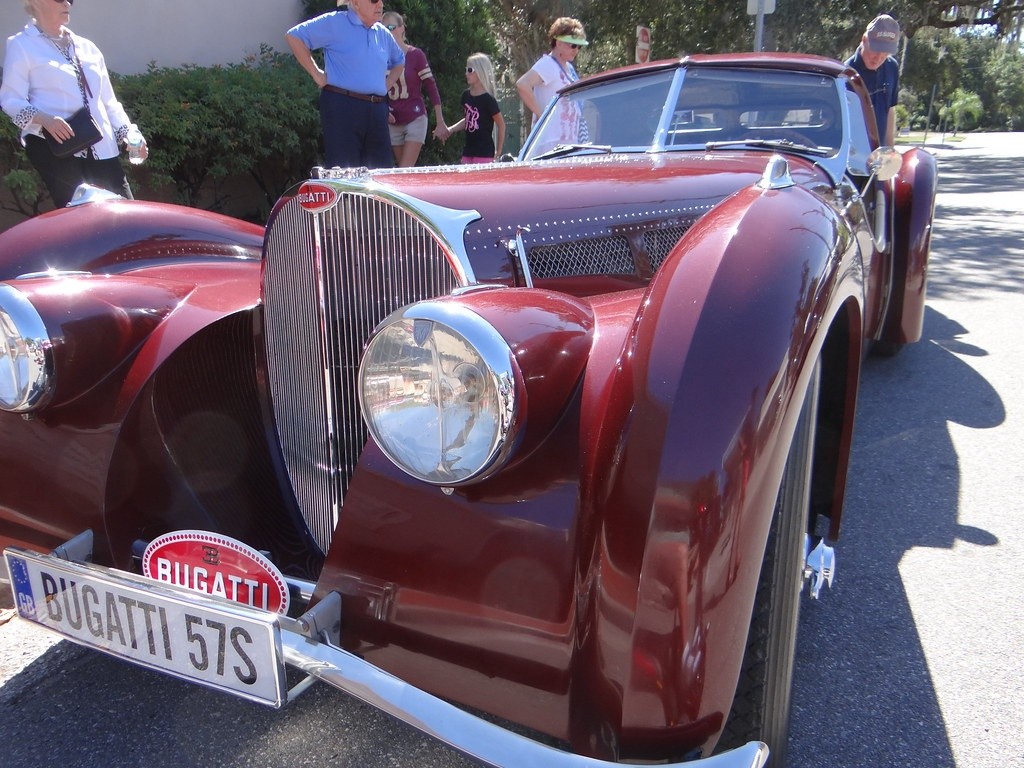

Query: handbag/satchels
<box><xmin>43</xmin><ymin>107</ymin><xmax>102</xmax><ymax>158</ymax></box>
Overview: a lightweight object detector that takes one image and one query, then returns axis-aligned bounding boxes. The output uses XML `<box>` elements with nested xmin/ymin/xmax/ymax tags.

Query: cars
<box><xmin>1</xmin><ymin>53</ymin><xmax>940</xmax><ymax>768</ymax></box>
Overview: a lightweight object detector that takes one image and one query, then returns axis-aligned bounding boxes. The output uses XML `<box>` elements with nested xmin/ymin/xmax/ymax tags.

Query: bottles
<box><xmin>126</xmin><ymin>124</ymin><xmax>144</xmax><ymax>165</ymax></box>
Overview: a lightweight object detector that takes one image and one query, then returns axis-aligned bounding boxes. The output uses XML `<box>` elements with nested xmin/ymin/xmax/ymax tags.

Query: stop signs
<box><xmin>635</xmin><ymin>26</ymin><xmax>651</xmax><ymax>64</ymax></box>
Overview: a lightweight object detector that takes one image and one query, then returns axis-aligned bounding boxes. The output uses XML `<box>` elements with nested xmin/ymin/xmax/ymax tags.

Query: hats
<box><xmin>553</xmin><ymin>35</ymin><xmax>589</xmax><ymax>46</ymax></box>
<box><xmin>867</xmin><ymin>14</ymin><xmax>900</xmax><ymax>54</ymax></box>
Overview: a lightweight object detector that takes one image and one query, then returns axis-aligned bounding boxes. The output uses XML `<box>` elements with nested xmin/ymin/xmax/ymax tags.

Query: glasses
<box><xmin>54</xmin><ymin>0</ymin><xmax>73</xmax><ymax>5</ymax></box>
<box><xmin>387</xmin><ymin>24</ymin><xmax>403</xmax><ymax>31</ymax></box>
<box><xmin>567</xmin><ymin>44</ymin><xmax>582</xmax><ymax>49</ymax></box>
<box><xmin>465</xmin><ymin>67</ymin><xmax>475</xmax><ymax>74</ymax></box>
<box><xmin>371</xmin><ymin>0</ymin><xmax>383</xmax><ymax>4</ymax></box>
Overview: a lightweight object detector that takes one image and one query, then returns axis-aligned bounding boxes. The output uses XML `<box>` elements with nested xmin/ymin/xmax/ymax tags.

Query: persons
<box><xmin>845</xmin><ymin>14</ymin><xmax>900</xmax><ymax>147</ymax></box>
<box><xmin>432</xmin><ymin>52</ymin><xmax>505</xmax><ymax>163</ymax></box>
<box><xmin>381</xmin><ymin>11</ymin><xmax>449</xmax><ymax>168</ymax></box>
<box><xmin>0</xmin><ymin>0</ymin><xmax>148</xmax><ymax>210</ymax></box>
<box><xmin>515</xmin><ymin>17</ymin><xmax>589</xmax><ymax>157</ymax></box>
<box><xmin>285</xmin><ymin>0</ymin><xmax>405</xmax><ymax>168</ymax></box>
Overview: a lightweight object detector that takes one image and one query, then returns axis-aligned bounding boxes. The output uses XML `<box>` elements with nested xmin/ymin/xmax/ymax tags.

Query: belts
<box><xmin>323</xmin><ymin>85</ymin><xmax>388</xmax><ymax>103</ymax></box>
